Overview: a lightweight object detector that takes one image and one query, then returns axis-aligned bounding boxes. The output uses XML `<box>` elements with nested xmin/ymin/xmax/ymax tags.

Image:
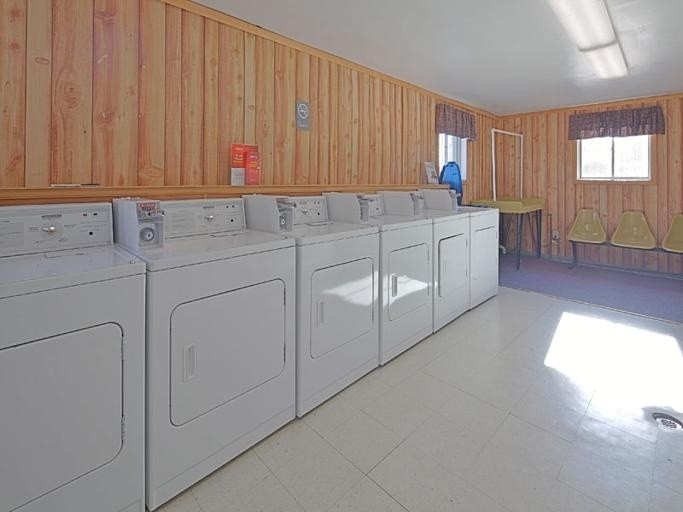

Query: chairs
<box><xmin>566</xmin><ymin>209</ymin><xmax>683</xmax><ymax>269</ymax></box>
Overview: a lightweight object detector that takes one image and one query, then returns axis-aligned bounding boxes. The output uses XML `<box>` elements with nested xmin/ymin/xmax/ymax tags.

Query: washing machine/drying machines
<box><xmin>113</xmin><ymin>187</ymin><xmax>502</xmax><ymax>512</ymax></box>
<box><xmin>3</xmin><ymin>187</ymin><xmax>149</xmax><ymax>512</ymax></box>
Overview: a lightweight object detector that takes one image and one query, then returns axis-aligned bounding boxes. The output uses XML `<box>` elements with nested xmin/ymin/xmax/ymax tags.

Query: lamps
<box><xmin>547</xmin><ymin>0</ymin><xmax>615</xmax><ymax>50</ymax></box>
<box><xmin>580</xmin><ymin>42</ymin><xmax>629</xmax><ymax>80</ymax></box>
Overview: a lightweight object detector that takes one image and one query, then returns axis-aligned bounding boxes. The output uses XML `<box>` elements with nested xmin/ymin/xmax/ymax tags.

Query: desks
<box><xmin>471</xmin><ymin>196</ymin><xmax>545</xmax><ymax>271</ymax></box>
